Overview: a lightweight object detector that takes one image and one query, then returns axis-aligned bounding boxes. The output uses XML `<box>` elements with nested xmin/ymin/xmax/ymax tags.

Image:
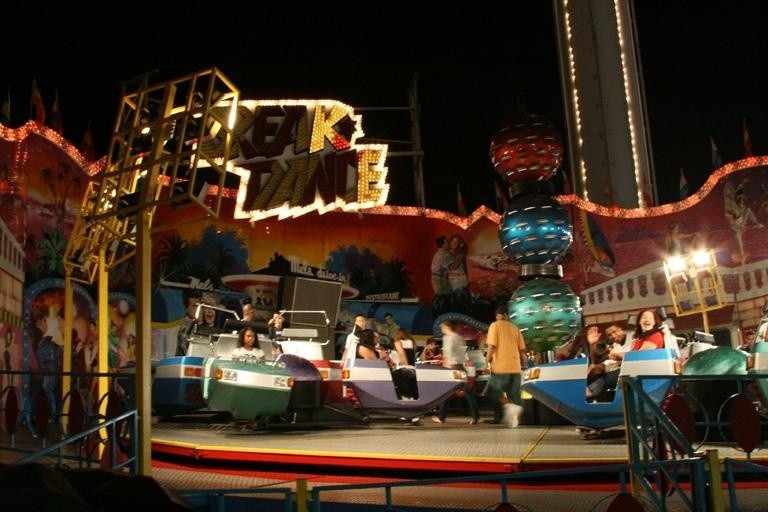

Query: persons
<box><xmin>176</xmin><ymin>290</ymin><xmax>238</xmax><ymax>356</ymax></box>
<box><xmin>555</xmin><ymin>308</ymin><xmax>667</xmax><ymax>401</ymax></box>
<box><xmin>232</xmin><ymin>326</ymin><xmax>265</xmax><ymax>364</ymax></box>
<box><xmin>31</xmin><ymin>299</ymin><xmax>128</xmax><ymax>430</ymax></box>
<box><xmin>378</xmin><ymin>312</ymin><xmax>400</xmax><ymax>342</ymax></box>
<box><xmin>268</xmin><ymin>312</ymin><xmax>289</xmax><ymax>341</ymax></box>
<box><xmin>341</xmin><ymin>315</ymin><xmax>480</xmax><ymax>425</ymax></box>
<box><xmin>481</xmin><ymin>310</ymin><xmax>529</xmax><ymax>429</ymax></box>
<box><xmin>240</xmin><ymin>304</ymin><xmax>258</xmax><ymax>324</ymax></box>
<box><xmin>430</xmin><ymin>235</ymin><xmax>468</xmax><ymax>295</ymax></box>
<box><xmin>736</xmin><ymin>331</ymin><xmax>756</xmax><ymax>353</ymax></box>
